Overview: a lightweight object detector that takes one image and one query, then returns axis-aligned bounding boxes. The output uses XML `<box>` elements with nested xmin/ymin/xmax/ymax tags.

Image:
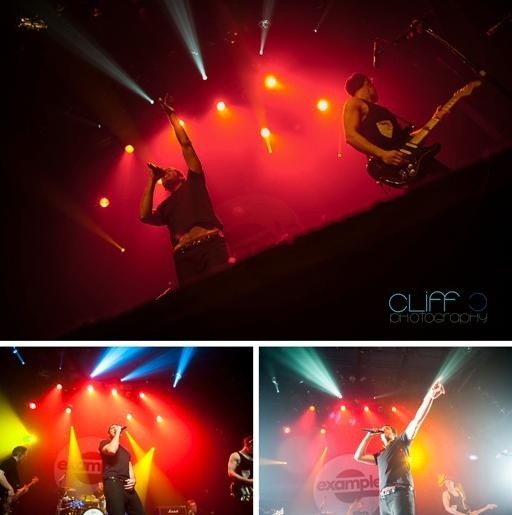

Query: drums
<box><xmin>82</xmin><ymin>508</ymin><xmax>104</xmax><ymax>515</ymax></box>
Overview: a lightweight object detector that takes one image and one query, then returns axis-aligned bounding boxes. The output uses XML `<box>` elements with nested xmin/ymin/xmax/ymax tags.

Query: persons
<box><xmin>341</xmin><ymin>64</ymin><xmax>447</xmax><ymax>186</ymax></box>
<box><xmin>353</xmin><ymin>378</ymin><xmax>446</xmax><ymax>515</ymax></box>
<box><xmin>136</xmin><ymin>93</ymin><xmax>231</xmax><ymax>284</ymax></box>
<box><xmin>224</xmin><ymin>435</ymin><xmax>253</xmax><ymax>511</ymax></box>
<box><xmin>439</xmin><ymin>476</ymin><xmax>501</xmax><ymax>515</ymax></box>
<box><xmin>1</xmin><ymin>442</ymin><xmax>28</xmax><ymax>514</ymax></box>
<box><xmin>97</xmin><ymin>421</ymin><xmax>146</xmax><ymax>514</ymax></box>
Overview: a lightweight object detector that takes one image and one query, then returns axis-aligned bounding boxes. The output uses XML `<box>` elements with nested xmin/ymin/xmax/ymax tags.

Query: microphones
<box><xmin>370</xmin><ymin>40</ymin><xmax>381</xmax><ymax>69</ymax></box>
<box><xmin>148</xmin><ymin>161</ymin><xmax>164</xmax><ymax>176</ymax></box>
<box><xmin>361</xmin><ymin>428</ymin><xmax>385</xmax><ymax>434</ymax></box>
<box><xmin>114</xmin><ymin>426</ymin><xmax>127</xmax><ymax>433</ymax></box>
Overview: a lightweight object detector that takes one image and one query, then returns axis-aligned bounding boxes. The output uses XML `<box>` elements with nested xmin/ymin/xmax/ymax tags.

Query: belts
<box><xmin>174</xmin><ymin>229</ymin><xmax>223</xmax><ymax>258</ymax></box>
<box><xmin>380</xmin><ymin>485</ymin><xmax>413</xmax><ymax>499</ymax></box>
<box><xmin>107</xmin><ymin>476</ymin><xmax>126</xmax><ymax>485</ymax></box>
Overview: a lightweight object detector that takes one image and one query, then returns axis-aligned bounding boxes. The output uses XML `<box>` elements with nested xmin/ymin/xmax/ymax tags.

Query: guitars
<box><xmin>366</xmin><ymin>81</ymin><xmax>481</xmax><ymax>187</ymax></box>
<box><xmin>0</xmin><ymin>477</ymin><xmax>40</xmax><ymax>515</ymax></box>
<box><xmin>231</xmin><ymin>483</ymin><xmax>253</xmax><ymax>502</ymax></box>
<box><xmin>466</xmin><ymin>504</ymin><xmax>498</xmax><ymax>515</ymax></box>
